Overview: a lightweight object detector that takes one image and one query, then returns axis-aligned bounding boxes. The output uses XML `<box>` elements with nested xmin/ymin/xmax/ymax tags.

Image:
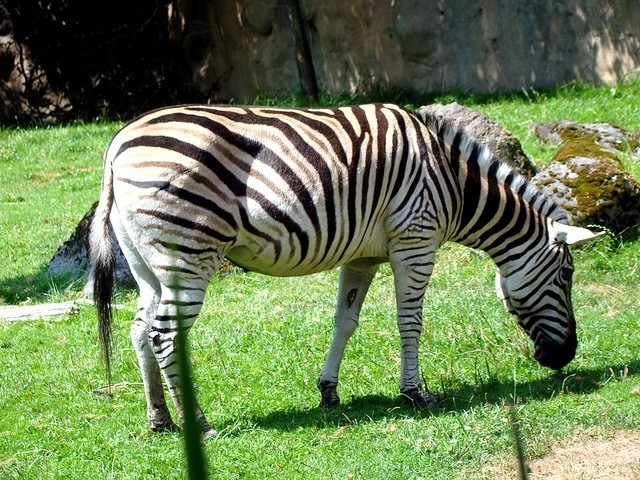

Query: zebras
<box><xmin>90</xmin><ymin>103</ymin><xmax>605</xmax><ymax>440</ymax></box>
